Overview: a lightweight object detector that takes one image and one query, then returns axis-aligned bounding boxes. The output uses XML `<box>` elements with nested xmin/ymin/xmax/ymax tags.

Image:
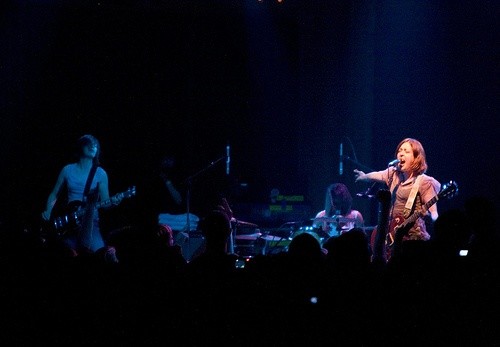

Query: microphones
<box><xmin>339</xmin><ymin>143</ymin><xmax>344</xmax><ymax>174</ymax></box>
<box><xmin>225</xmin><ymin>146</ymin><xmax>230</xmax><ymax>173</ymax></box>
<box><xmin>286</xmin><ymin>221</ymin><xmax>300</xmax><ymax>225</ymax></box>
<box><xmin>388</xmin><ymin>159</ymin><xmax>400</xmax><ymax>165</ymax></box>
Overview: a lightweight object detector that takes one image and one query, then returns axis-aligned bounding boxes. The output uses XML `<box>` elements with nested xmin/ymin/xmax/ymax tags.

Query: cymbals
<box><xmin>237</xmin><ymin>234</ymin><xmax>286</xmax><ymax>243</ymax></box>
<box><xmin>229</xmin><ymin>216</ymin><xmax>259</xmax><ymax>226</ymax></box>
<box><xmin>309</xmin><ymin>217</ymin><xmax>358</xmax><ymax>222</ymax></box>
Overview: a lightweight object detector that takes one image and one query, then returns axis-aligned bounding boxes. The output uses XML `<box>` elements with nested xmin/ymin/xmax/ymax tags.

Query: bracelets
<box><xmin>377</xmin><ymin>210</ymin><xmax>389</xmax><ymax>216</ymax></box>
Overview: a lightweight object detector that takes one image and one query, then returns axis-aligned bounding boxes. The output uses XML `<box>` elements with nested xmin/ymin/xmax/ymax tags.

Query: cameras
<box><xmin>459</xmin><ymin>249</ymin><xmax>469</xmax><ymax>256</ymax></box>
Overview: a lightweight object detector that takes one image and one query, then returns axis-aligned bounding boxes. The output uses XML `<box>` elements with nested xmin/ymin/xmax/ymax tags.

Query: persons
<box><xmin>41</xmin><ymin>134</ymin><xmax>122</xmax><ymax>255</ymax></box>
<box><xmin>353</xmin><ymin>137</ymin><xmax>441</xmax><ymax>259</ymax></box>
<box><xmin>312</xmin><ymin>183</ymin><xmax>368</xmax><ymax>239</ymax></box>
<box><xmin>0</xmin><ymin>189</ymin><xmax>500</xmax><ymax>347</ymax></box>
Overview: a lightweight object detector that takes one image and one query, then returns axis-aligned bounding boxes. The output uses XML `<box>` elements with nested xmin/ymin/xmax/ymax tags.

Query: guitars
<box><xmin>368</xmin><ymin>175</ymin><xmax>456</xmax><ymax>266</ymax></box>
<box><xmin>36</xmin><ymin>182</ymin><xmax>137</xmax><ymax>243</ymax></box>
